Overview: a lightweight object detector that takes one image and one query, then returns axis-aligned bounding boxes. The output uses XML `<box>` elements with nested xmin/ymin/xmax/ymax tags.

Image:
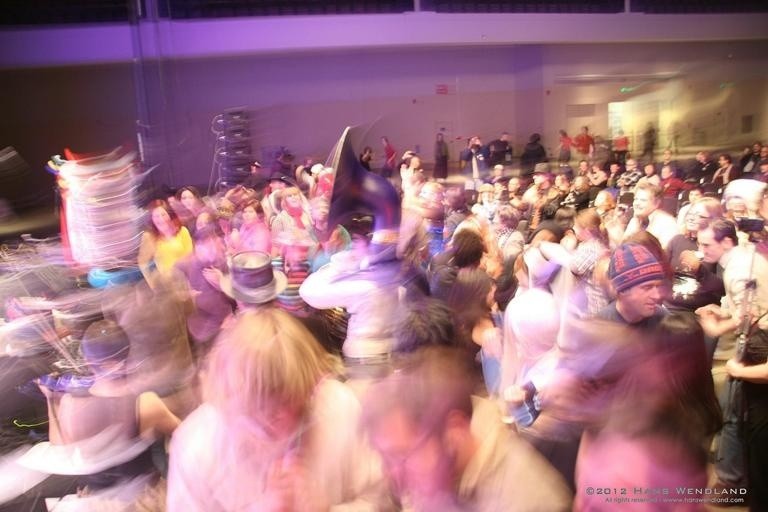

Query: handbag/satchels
<box><xmin>738</xmin><ymin>322</ymin><xmax>768</xmax><ymax>364</ymax></box>
<box><xmin>667</xmin><ymin>273</ymin><xmax>713</xmax><ymax>308</ymax></box>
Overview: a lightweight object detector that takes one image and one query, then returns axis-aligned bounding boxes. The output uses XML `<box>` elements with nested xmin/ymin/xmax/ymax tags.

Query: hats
<box><xmin>77</xmin><ymin>320</ymin><xmax>129</xmax><ymax>360</ymax></box>
<box><xmin>529</xmin><ymin>163</ymin><xmax>552</xmax><ymax>175</ymax></box>
<box><xmin>607</xmin><ymin>243</ymin><xmax>666</xmax><ymax>293</ymax></box>
<box><xmin>220</xmin><ymin>250</ymin><xmax>287</xmax><ymax>305</ymax></box>
<box><xmin>266</xmin><ymin>171</ymin><xmax>295</xmax><ymax>186</ymax></box>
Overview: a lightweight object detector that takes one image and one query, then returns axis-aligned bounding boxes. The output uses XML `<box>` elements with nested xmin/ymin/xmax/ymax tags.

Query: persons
<box><xmin>35</xmin><ymin>126</ymin><xmax>768</xmax><ymax>512</ymax></box>
